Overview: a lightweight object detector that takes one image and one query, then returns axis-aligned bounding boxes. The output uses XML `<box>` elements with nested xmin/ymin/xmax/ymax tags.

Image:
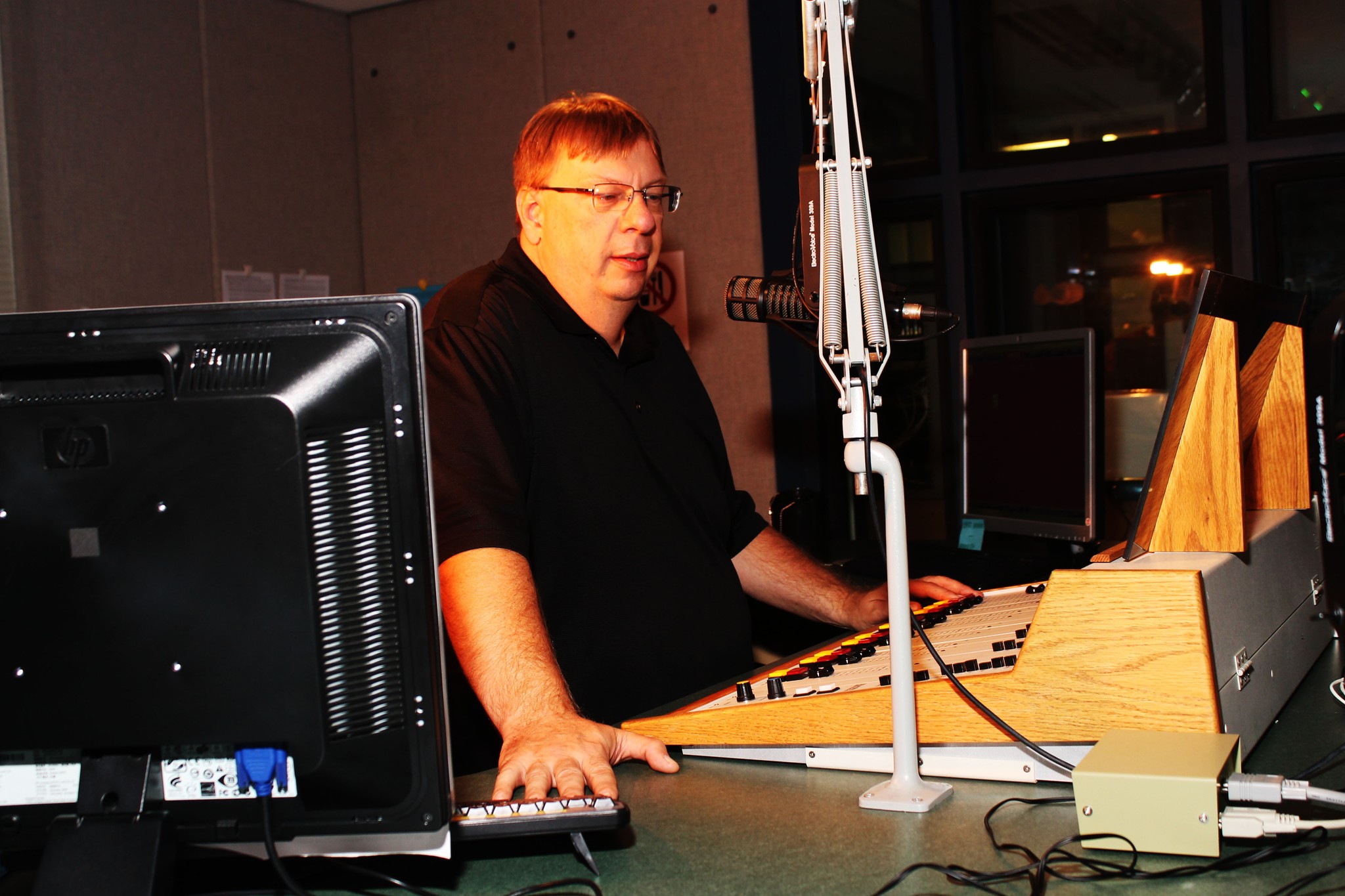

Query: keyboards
<box><xmin>453</xmin><ymin>797</ymin><xmax>629</xmax><ymax>850</ymax></box>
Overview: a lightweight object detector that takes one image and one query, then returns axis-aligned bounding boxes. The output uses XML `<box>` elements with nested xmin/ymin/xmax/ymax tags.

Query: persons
<box><xmin>421</xmin><ymin>93</ymin><xmax>985</xmax><ymax>802</ymax></box>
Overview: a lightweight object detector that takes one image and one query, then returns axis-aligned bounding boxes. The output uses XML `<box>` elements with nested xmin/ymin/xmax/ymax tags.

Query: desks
<box><xmin>364</xmin><ymin>723</ymin><xmax>1345</xmax><ymax>896</ymax></box>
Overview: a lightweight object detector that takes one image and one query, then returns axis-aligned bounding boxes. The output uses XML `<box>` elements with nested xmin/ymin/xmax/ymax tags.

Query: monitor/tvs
<box><xmin>959</xmin><ymin>328</ymin><xmax>1097</xmax><ymax>543</ymax></box>
<box><xmin>0</xmin><ymin>293</ymin><xmax>455</xmax><ymax>895</ymax></box>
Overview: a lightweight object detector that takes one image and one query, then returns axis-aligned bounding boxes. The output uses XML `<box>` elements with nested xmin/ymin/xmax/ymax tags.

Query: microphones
<box><xmin>723</xmin><ymin>274</ymin><xmax>954</xmax><ymax>337</ymax></box>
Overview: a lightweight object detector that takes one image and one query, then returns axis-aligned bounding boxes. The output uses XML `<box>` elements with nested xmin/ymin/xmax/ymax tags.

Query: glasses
<box><xmin>533</xmin><ymin>182</ymin><xmax>684</xmax><ymax>213</ymax></box>
